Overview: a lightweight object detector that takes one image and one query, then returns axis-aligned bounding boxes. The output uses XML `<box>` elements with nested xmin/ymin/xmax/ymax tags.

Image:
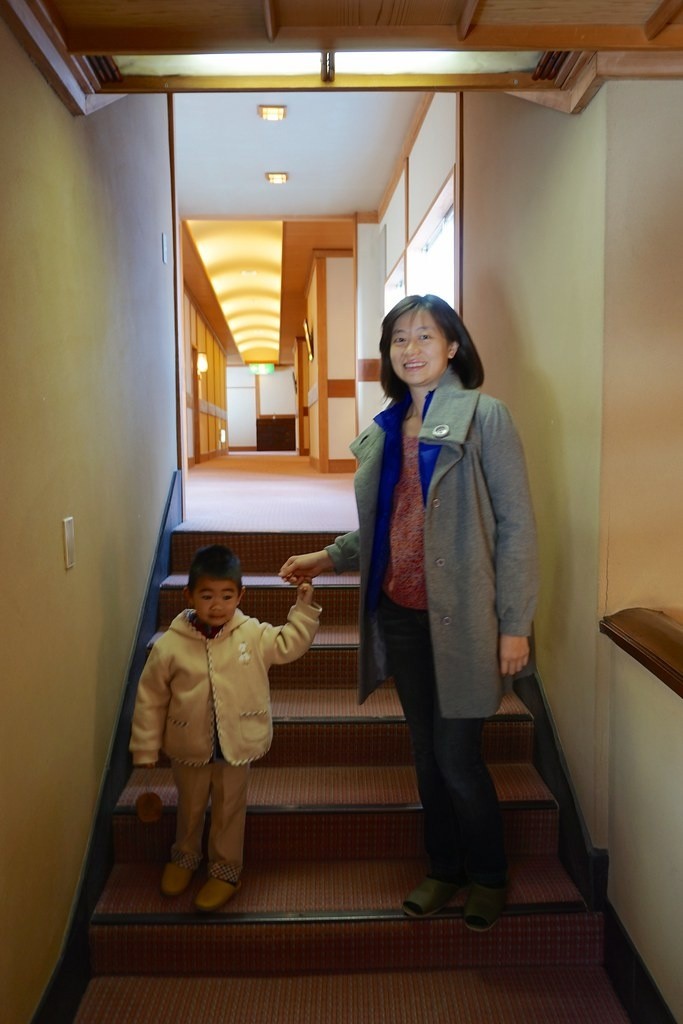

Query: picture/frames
<box><xmin>303</xmin><ymin>318</ymin><xmax>314</xmax><ymax>361</ymax></box>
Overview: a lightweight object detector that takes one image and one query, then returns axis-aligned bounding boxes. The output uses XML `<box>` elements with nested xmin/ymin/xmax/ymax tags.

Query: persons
<box><xmin>277</xmin><ymin>293</ymin><xmax>542</xmax><ymax>932</ymax></box>
<box><xmin>128</xmin><ymin>545</ymin><xmax>322</xmax><ymax>909</ymax></box>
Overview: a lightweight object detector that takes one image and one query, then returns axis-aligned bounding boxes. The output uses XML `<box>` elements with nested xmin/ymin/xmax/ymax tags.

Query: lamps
<box><xmin>267</xmin><ymin>173</ymin><xmax>288</xmax><ymax>184</ymax></box>
<box><xmin>258</xmin><ymin>104</ymin><xmax>285</xmax><ymax>120</ymax></box>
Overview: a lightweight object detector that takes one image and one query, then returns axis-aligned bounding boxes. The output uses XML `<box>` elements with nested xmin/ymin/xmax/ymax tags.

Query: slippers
<box><xmin>162</xmin><ymin>852</ymin><xmax>204</xmax><ymax>895</ymax></box>
<box><xmin>194</xmin><ymin>879</ymin><xmax>241</xmax><ymax>912</ymax></box>
<box><xmin>464</xmin><ymin>881</ymin><xmax>506</xmax><ymax>932</ymax></box>
<box><xmin>402</xmin><ymin>877</ymin><xmax>460</xmax><ymax>918</ymax></box>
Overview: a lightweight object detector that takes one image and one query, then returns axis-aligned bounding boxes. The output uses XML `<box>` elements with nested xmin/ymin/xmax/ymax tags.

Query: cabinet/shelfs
<box><xmin>257</xmin><ymin>413</ymin><xmax>295</xmax><ymax>450</ymax></box>
